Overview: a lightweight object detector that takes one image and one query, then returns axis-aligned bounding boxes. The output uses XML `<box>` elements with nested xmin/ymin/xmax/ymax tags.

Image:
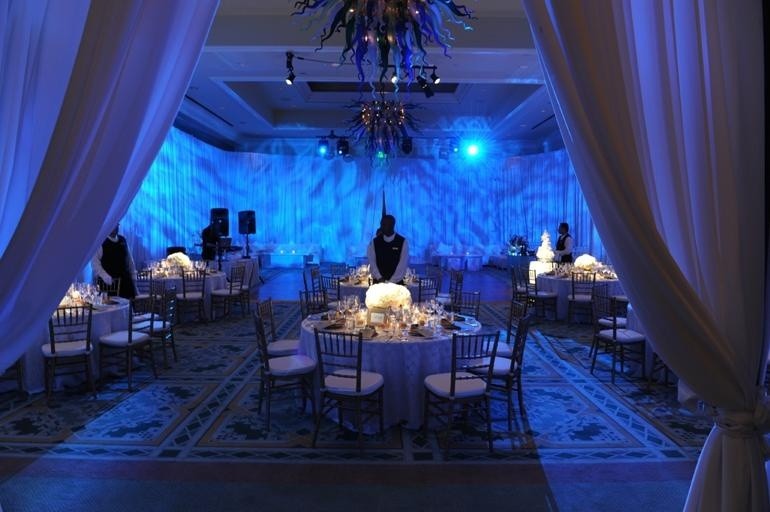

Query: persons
<box><xmin>202</xmin><ymin>217</ymin><xmax>223</xmax><ymax>261</ymax></box>
<box><xmin>368</xmin><ymin>215</ymin><xmax>408</xmax><ymax>284</ymax></box>
<box><xmin>91</xmin><ymin>222</ymin><xmax>139</xmax><ymax>313</ymax></box>
<box><xmin>554</xmin><ymin>222</ymin><xmax>575</xmax><ymax>263</ymax></box>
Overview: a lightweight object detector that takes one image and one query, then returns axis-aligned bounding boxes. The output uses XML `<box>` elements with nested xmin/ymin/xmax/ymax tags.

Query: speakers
<box><xmin>210</xmin><ymin>207</ymin><xmax>229</xmax><ymax>237</ymax></box>
<box><xmin>166</xmin><ymin>246</ymin><xmax>186</xmax><ymax>258</ymax></box>
<box><xmin>238</xmin><ymin>210</ymin><xmax>256</xmax><ymax>235</ymax></box>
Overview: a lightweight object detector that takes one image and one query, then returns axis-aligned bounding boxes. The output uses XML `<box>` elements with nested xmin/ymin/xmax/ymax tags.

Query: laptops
<box><xmin>217</xmin><ymin>238</ymin><xmax>232</xmax><ymax>247</ymax></box>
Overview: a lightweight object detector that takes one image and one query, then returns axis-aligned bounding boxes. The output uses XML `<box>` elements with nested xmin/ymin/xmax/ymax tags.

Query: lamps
<box><xmin>289</xmin><ymin>0</ymin><xmax>474</xmax><ymax>95</ymax></box>
<box><xmin>314</xmin><ymin>130</ymin><xmax>414</xmax><ymax>158</ymax></box>
<box><xmin>340</xmin><ymin>95</ymin><xmax>423</xmax><ymax>155</ymax></box>
<box><xmin>280</xmin><ymin>51</ymin><xmax>440</xmax><ymax>90</ymax></box>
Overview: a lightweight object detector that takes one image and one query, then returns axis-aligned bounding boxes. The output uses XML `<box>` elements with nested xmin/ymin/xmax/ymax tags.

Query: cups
<box><xmin>146</xmin><ymin>258</ymin><xmax>207</xmax><ymax>276</ymax></box>
<box><xmin>65</xmin><ymin>282</ymin><xmax>108</xmax><ymax>311</ymax></box>
<box><xmin>327</xmin><ymin>294</ymin><xmax>453</xmax><ymax>343</ymax></box>
<box><xmin>349</xmin><ymin>265</ymin><xmax>416</xmax><ymax>284</ymax></box>
<box><xmin>555</xmin><ymin>262</ymin><xmax>613</xmax><ymax>277</ymax></box>
